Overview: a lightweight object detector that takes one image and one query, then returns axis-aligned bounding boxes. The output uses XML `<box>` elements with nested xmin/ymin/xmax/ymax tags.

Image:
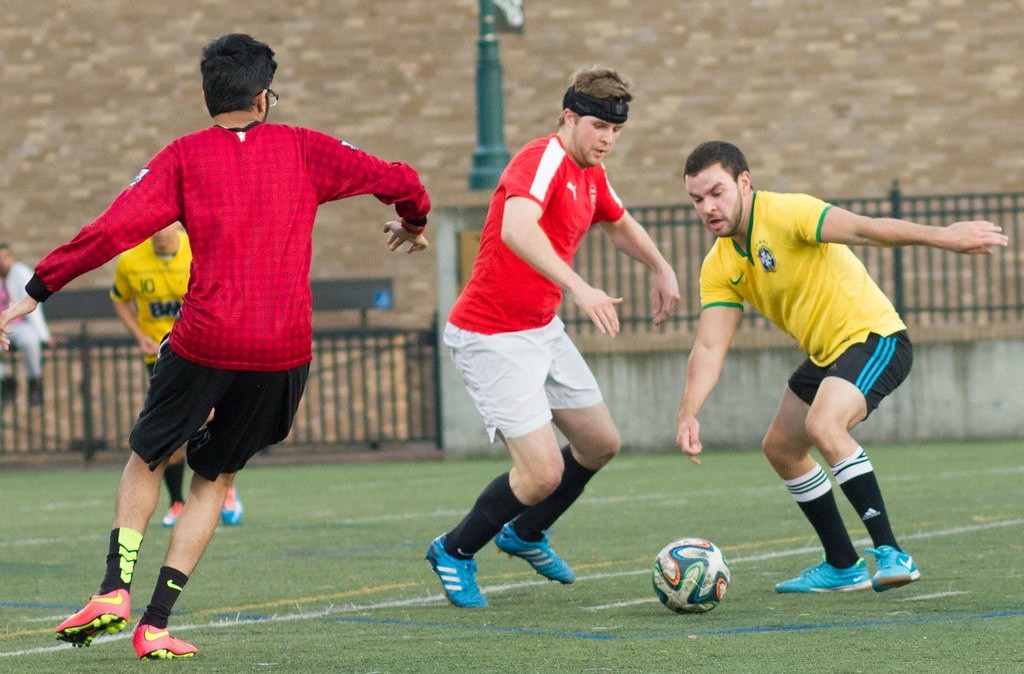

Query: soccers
<box><xmin>649</xmin><ymin>535</ymin><xmax>732</xmax><ymax>615</ymax></box>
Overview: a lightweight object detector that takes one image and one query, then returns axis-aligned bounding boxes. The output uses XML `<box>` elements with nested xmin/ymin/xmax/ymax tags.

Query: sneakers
<box><xmin>773</xmin><ymin>552</ymin><xmax>872</xmax><ymax>598</ymax></box>
<box><xmin>51</xmin><ymin>586</ymin><xmax>132</xmax><ymax>649</ymax></box>
<box><xmin>221</xmin><ymin>486</ymin><xmax>240</xmax><ymax>523</ymax></box>
<box><xmin>162</xmin><ymin>503</ymin><xmax>182</xmax><ymax>520</ymax></box>
<box><xmin>868</xmin><ymin>542</ymin><xmax>922</xmax><ymax>593</ymax></box>
<box><xmin>132</xmin><ymin>623</ymin><xmax>199</xmax><ymax>664</ymax></box>
<box><xmin>421</xmin><ymin>532</ymin><xmax>490</xmax><ymax>608</ymax></box>
<box><xmin>495</xmin><ymin>522</ymin><xmax>580</xmax><ymax>584</ymax></box>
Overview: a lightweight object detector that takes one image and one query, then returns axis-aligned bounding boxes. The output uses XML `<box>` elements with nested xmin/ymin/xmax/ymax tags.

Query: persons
<box><xmin>677</xmin><ymin>141</ymin><xmax>1009</xmax><ymax>594</ymax></box>
<box><xmin>426</xmin><ymin>70</ymin><xmax>681</xmax><ymax>607</ymax></box>
<box><xmin>0</xmin><ymin>32</ymin><xmax>430</xmax><ymax>661</ymax></box>
<box><xmin>108</xmin><ymin>222</ymin><xmax>244</xmax><ymax>527</ymax></box>
<box><xmin>0</xmin><ymin>242</ymin><xmax>69</xmax><ymax>406</ymax></box>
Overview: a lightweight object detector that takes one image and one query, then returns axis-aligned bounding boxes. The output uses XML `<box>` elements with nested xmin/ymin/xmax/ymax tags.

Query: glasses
<box><xmin>252</xmin><ymin>88</ymin><xmax>282</xmax><ymax>106</ymax></box>
